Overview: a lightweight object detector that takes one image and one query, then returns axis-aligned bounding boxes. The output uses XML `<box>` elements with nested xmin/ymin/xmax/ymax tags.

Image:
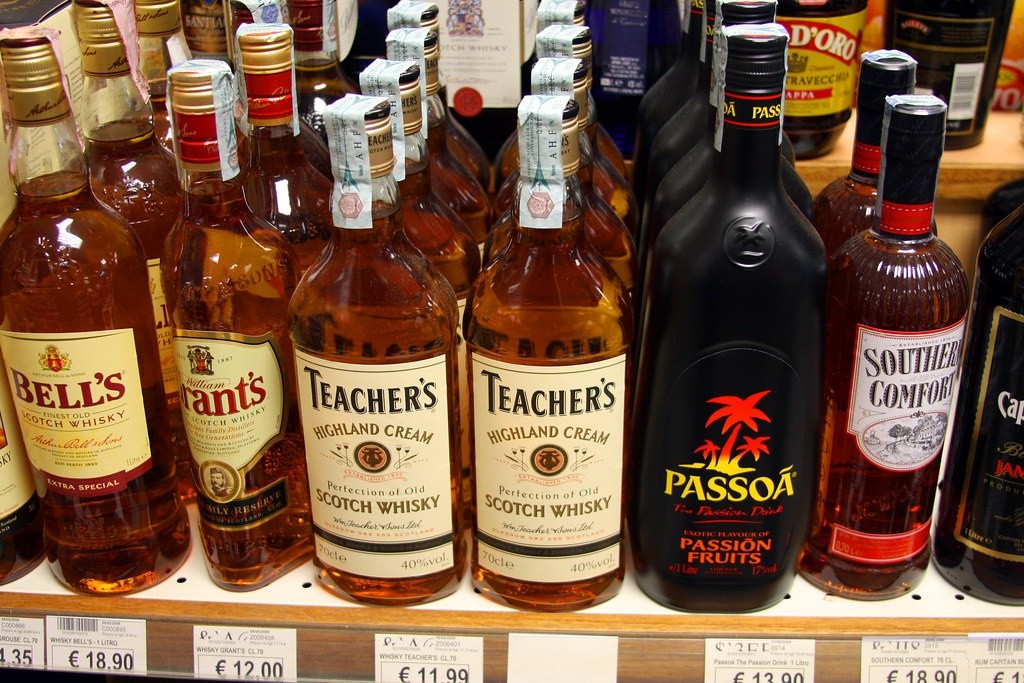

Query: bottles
<box><xmin>0</xmin><ymin>0</ymin><xmax>1024</xmax><ymax>613</ymax></box>
